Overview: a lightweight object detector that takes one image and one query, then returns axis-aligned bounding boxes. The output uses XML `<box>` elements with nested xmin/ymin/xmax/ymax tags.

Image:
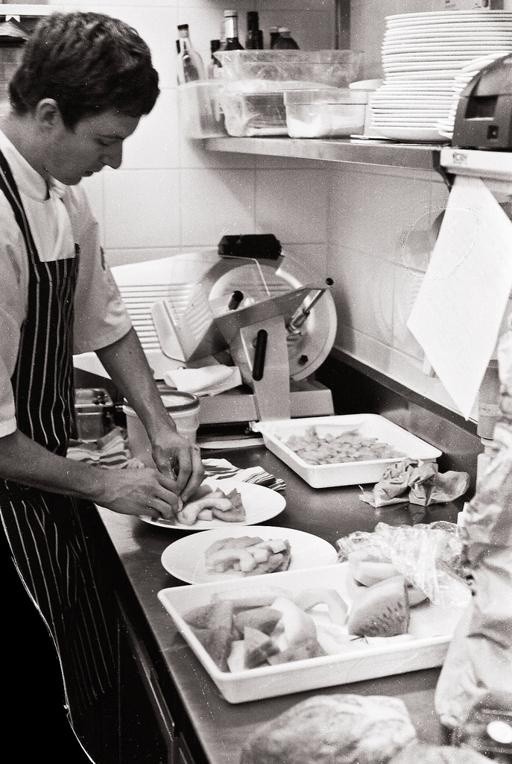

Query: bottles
<box><xmin>173</xmin><ymin>9</ymin><xmax>299</xmax><ymax>132</ymax></box>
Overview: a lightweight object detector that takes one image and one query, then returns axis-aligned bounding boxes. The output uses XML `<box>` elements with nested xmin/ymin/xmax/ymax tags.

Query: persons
<box><xmin>0</xmin><ymin>11</ymin><xmax>205</xmax><ymax>764</ymax></box>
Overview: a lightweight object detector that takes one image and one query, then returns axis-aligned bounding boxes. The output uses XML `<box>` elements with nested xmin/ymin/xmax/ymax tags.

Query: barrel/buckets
<box><xmin>121</xmin><ymin>392</ymin><xmax>202</xmax><ymax>472</ymax></box>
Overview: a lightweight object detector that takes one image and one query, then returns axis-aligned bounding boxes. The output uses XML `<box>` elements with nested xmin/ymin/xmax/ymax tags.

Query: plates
<box><xmin>139</xmin><ymin>480</ymin><xmax>340</xmax><ymax>587</ymax></box>
<box><xmin>369</xmin><ymin>10</ymin><xmax>511</xmax><ymax>142</ymax></box>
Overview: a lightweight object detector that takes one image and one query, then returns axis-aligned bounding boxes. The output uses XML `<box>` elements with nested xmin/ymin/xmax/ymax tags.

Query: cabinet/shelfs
<box><xmin>115</xmin><ymin>596</ymin><xmax>201</xmax><ymax>764</ymax></box>
